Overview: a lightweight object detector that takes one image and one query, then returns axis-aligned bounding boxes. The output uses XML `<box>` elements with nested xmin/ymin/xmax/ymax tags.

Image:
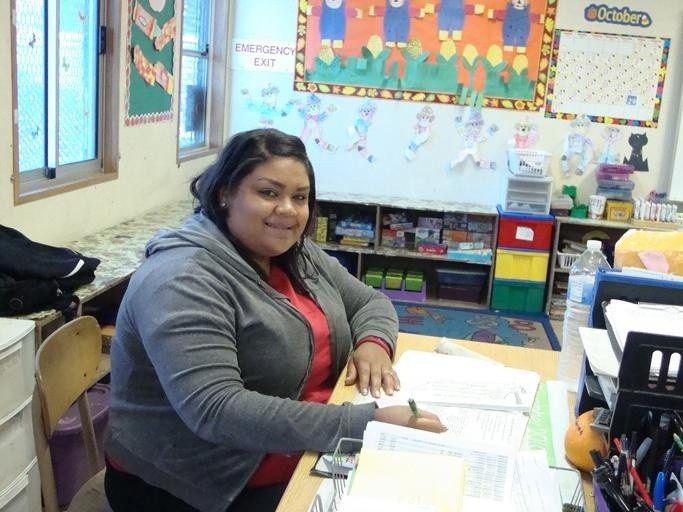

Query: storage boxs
<box><xmin>490</xmin><ymin>204</ymin><xmax>554</xmax><ymax>313</ymax></box>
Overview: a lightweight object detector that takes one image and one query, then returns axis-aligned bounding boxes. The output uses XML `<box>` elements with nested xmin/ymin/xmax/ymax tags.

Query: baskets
<box><xmin>309</xmin><ymin>437</ymin><xmax>586</xmax><ymax>512</ymax></box>
<box><xmin>507</xmin><ymin>148</ymin><xmax>553</xmax><ymax>177</ymax></box>
<box><xmin>557</xmin><ymin>250</ymin><xmax>607</xmax><ymax>269</ymax></box>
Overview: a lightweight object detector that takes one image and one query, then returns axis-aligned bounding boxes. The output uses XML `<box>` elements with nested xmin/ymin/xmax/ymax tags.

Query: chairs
<box><xmin>34</xmin><ymin>315</ymin><xmax>115</xmax><ymax>512</ymax></box>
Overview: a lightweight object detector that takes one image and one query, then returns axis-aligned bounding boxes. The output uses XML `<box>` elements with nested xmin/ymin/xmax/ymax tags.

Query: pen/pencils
<box><xmin>589</xmin><ymin>431</ymin><xmax>683</xmax><ymax>512</ymax></box>
<box><xmin>408</xmin><ymin>399</ymin><xmax>420</xmax><ymax>417</ymax></box>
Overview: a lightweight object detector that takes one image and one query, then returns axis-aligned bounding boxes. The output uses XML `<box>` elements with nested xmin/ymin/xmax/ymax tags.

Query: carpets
<box><xmin>391</xmin><ymin>300</ymin><xmax>561</xmax><ymax>352</ymax></box>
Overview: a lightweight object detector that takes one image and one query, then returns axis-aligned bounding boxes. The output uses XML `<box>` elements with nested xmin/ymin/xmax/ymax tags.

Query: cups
<box><xmin>588</xmin><ymin>194</ymin><xmax>607</xmax><ymax>220</ymax></box>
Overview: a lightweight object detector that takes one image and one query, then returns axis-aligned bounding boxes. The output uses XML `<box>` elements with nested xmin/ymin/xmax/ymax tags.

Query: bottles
<box><xmin>556</xmin><ymin>237</ymin><xmax>613</xmax><ymax>395</ymax></box>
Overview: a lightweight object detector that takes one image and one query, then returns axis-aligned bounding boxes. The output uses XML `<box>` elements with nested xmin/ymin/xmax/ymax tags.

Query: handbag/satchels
<box><xmin>0</xmin><ymin>224</ymin><xmax>100</xmax><ymax>318</ymax></box>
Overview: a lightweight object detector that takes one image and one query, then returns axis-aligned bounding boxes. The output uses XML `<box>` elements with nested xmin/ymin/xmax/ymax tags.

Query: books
<box><xmin>311</xmin><ymin>348</ymin><xmax>586</xmax><ymax>512</ymax></box>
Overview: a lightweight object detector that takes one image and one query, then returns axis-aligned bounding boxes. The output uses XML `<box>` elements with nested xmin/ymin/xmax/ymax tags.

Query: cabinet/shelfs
<box><xmin>0</xmin><ymin>316</ymin><xmax>41</xmax><ymax>512</ymax></box>
<box><xmin>12</xmin><ymin>201</ymin><xmax>224</xmax><ymax>393</ymax></box>
<box><xmin>508</xmin><ymin>177</ymin><xmax>551</xmax><ymax>216</ymax></box>
<box><xmin>303</xmin><ymin>193</ymin><xmax>499</xmax><ymax>312</ymax></box>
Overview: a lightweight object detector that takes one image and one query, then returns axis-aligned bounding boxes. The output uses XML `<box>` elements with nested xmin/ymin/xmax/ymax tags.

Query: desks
<box><xmin>274</xmin><ymin>332</ymin><xmax>596</xmax><ymax>512</ymax></box>
<box><xmin>545</xmin><ymin>215</ymin><xmax>683</xmax><ymax>326</ymax></box>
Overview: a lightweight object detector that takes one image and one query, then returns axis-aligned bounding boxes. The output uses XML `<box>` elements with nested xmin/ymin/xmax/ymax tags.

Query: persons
<box><xmin>99</xmin><ymin>128</ymin><xmax>447</xmax><ymax>512</ymax></box>
